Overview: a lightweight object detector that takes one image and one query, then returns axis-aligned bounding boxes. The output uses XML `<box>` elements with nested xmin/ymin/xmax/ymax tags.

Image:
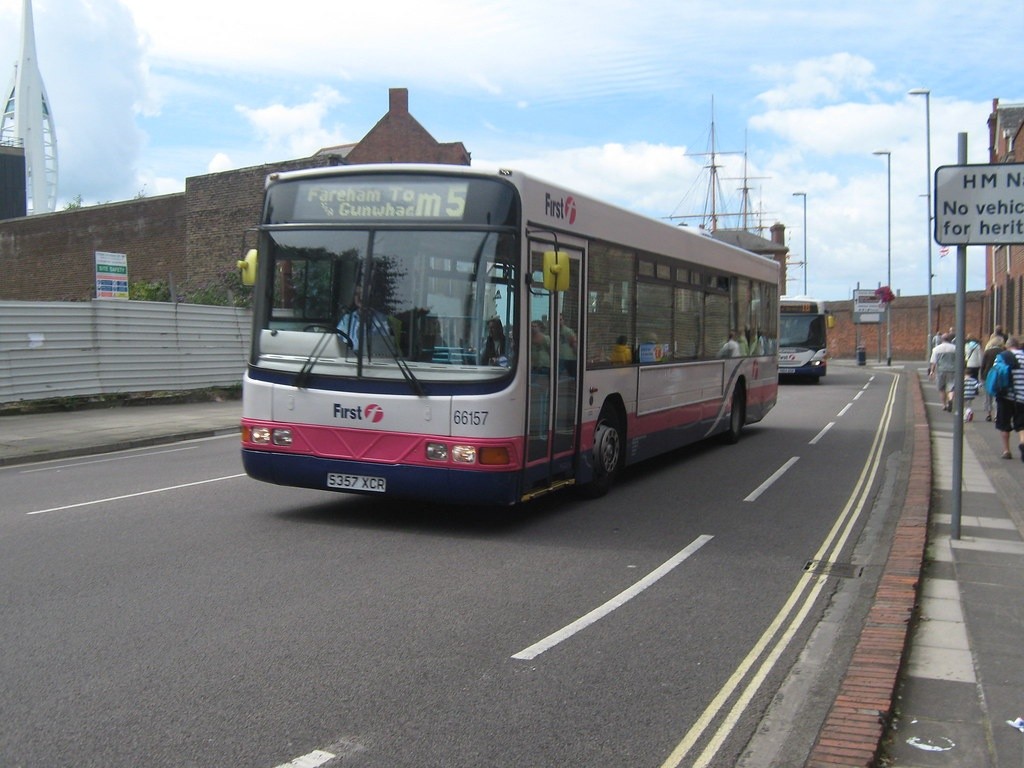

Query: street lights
<box><xmin>793</xmin><ymin>192</ymin><xmax>807</xmax><ymax>295</ymax></box>
<box><xmin>873</xmin><ymin>151</ymin><xmax>893</xmax><ymax>367</ymax></box>
<box><xmin>908</xmin><ymin>87</ymin><xmax>933</xmax><ymax>375</ymax></box>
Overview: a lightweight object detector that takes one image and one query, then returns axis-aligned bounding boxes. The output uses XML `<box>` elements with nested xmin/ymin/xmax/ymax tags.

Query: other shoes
<box><xmin>967</xmin><ymin>412</ymin><xmax>973</xmax><ymax>422</ymax></box>
<box><xmin>948</xmin><ymin>400</ymin><xmax>952</xmax><ymax>412</ymax></box>
<box><xmin>942</xmin><ymin>406</ymin><xmax>947</xmax><ymax>410</ymax></box>
<box><xmin>1002</xmin><ymin>451</ymin><xmax>1011</xmax><ymax>459</ymax></box>
<box><xmin>1018</xmin><ymin>443</ymin><xmax>1024</xmax><ymax>462</ymax></box>
<box><xmin>986</xmin><ymin>416</ymin><xmax>991</xmax><ymax>421</ymax></box>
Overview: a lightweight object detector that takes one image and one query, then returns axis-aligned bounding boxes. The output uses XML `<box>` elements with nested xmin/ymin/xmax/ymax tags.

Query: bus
<box><xmin>750</xmin><ymin>295</ymin><xmax>836</xmax><ymax>383</ymax></box>
<box><xmin>237</xmin><ymin>154</ymin><xmax>782</xmax><ymax>508</ymax></box>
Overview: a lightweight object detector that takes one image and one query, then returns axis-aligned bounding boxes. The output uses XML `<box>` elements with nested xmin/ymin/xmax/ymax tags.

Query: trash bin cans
<box><xmin>857</xmin><ymin>345</ymin><xmax>866</xmax><ymax>366</ymax></box>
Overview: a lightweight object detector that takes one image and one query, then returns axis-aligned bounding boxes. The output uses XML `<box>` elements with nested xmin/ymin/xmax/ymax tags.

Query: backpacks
<box><xmin>985</xmin><ymin>354</ymin><xmax>1012</xmax><ymax>396</ymax></box>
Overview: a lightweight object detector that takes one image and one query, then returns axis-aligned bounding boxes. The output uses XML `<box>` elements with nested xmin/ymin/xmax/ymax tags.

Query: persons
<box><xmin>717</xmin><ymin>325</ymin><xmax>774</xmax><ymax>359</ymax></box>
<box><xmin>336</xmin><ymin>284</ymin><xmax>399</xmax><ymax>359</ymax></box>
<box><xmin>612</xmin><ymin>336</ymin><xmax>632</xmax><ymax>364</ymax></box>
<box><xmin>980</xmin><ymin>325</ymin><xmax>1024</xmax><ymax>462</ymax></box>
<box><xmin>531</xmin><ymin>321</ymin><xmax>550</xmax><ymax>377</ymax></box>
<box><xmin>560</xmin><ymin>314</ymin><xmax>579</xmax><ymax>365</ymax></box>
<box><xmin>482</xmin><ymin>319</ymin><xmax>506</xmax><ymax>367</ymax></box>
<box><xmin>930</xmin><ymin>327</ymin><xmax>983</xmax><ymax>422</ymax></box>
